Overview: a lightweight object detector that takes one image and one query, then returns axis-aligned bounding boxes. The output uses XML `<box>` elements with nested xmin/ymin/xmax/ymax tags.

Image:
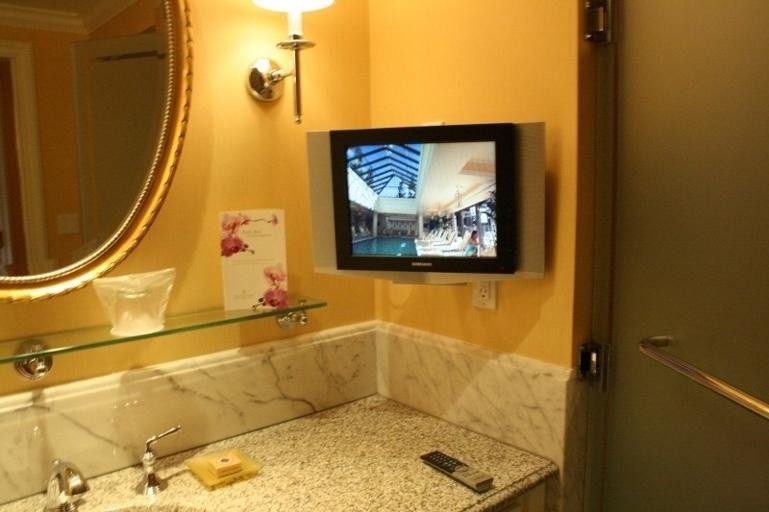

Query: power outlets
<box><xmin>472</xmin><ymin>278</ymin><xmax>497</xmax><ymax>310</ymax></box>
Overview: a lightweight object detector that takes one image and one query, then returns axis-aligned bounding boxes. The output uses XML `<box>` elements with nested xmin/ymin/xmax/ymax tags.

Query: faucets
<box><xmin>41</xmin><ymin>458</ymin><xmax>91</xmax><ymax>512</ymax></box>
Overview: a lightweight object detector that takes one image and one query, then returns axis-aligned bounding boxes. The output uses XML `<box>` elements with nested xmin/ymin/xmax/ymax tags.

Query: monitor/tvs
<box><xmin>307</xmin><ymin>121</ymin><xmax>545</xmax><ymax>282</ymax></box>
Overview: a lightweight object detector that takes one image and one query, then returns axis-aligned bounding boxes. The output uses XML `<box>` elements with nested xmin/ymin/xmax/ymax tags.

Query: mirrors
<box><xmin>2</xmin><ymin>1</ymin><xmax>194</xmax><ymax>306</ymax></box>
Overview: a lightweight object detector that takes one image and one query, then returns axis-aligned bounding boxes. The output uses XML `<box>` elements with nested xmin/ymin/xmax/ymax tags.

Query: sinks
<box><xmin>109</xmin><ymin>504</ymin><xmax>209</xmax><ymax>512</ymax></box>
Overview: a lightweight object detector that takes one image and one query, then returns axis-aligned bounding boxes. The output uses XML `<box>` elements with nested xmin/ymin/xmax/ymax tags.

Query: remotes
<box><xmin>420</xmin><ymin>450</ymin><xmax>493</xmax><ymax>493</ymax></box>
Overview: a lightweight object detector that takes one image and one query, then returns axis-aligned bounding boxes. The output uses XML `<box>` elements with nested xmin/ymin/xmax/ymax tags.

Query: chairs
<box><xmin>414</xmin><ymin>228</ymin><xmax>466</xmax><ymax>258</ymax></box>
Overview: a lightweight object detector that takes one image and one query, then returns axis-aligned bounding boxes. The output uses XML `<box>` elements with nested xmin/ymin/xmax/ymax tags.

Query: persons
<box><xmin>462</xmin><ymin>229</ymin><xmax>485</xmax><ymax>259</ymax></box>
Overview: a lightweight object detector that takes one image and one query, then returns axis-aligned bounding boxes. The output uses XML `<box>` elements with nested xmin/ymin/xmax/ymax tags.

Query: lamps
<box><xmin>244</xmin><ymin>0</ymin><xmax>336</xmax><ymax>126</ymax></box>
